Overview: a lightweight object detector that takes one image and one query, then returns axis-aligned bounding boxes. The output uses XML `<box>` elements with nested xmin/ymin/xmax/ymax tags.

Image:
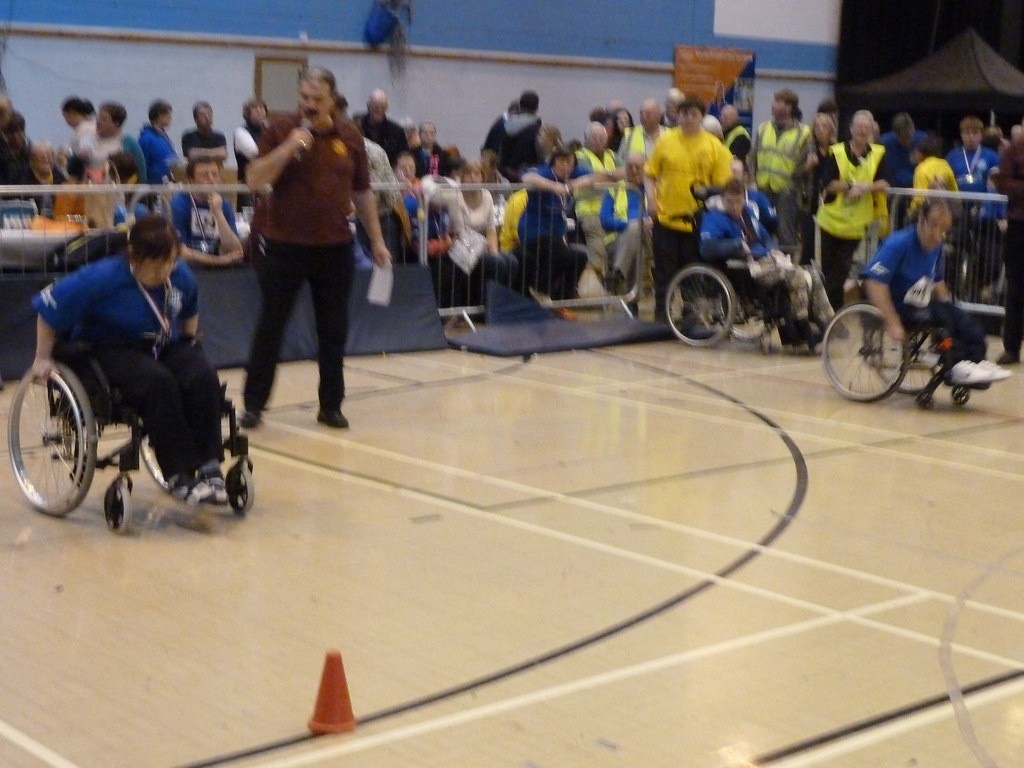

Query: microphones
<box><xmin>294</xmin><ymin>118</ymin><xmax>314</xmax><ymax>162</ymax></box>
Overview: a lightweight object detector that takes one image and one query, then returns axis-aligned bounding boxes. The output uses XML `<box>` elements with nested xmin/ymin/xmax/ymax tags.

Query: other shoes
<box><xmin>201</xmin><ymin>459</ymin><xmax>229</xmax><ymax>503</ymax></box>
<box><xmin>241</xmin><ymin>410</ymin><xmax>260</xmax><ymax>428</ymax></box>
<box><xmin>317</xmin><ymin>408</ymin><xmax>348</xmax><ymax>427</ymax></box>
<box><xmin>950</xmin><ymin>360</ymin><xmax>993</xmax><ymax>383</ymax></box>
<box><xmin>978</xmin><ymin>360</ymin><xmax>1012</xmax><ymax>380</ymax></box>
<box><xmin>168</xmin><ymin>474</ymin><xmax>214</xmax><ymax>506</ymax></box>
<box><xmin>793</xmin><ymin>318</ymin><xmax>811</xmax><ymax>340</ymax></box>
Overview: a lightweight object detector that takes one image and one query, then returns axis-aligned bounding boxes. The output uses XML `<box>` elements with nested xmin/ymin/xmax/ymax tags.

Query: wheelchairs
<box><xmin>8</xmin><ymin>234</ymin><xmax>256</xmax><ymax>536</ymax></box>
<box><xmin>665</xmin><ymin>207</ymin><xmax>825</xmax><ymax>357</ymax></box>
<box><xmin>818</xmin><ymin>274</ymin><xmax>994</xmax><ymax>411</ymax></box>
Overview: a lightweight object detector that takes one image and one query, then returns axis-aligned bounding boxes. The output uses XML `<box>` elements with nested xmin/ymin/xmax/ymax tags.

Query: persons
<box><xmin>238</xmin><ymin>66</ymin><xmax>394</xmax><ymax>428</ymax></box>
<box><xmin>30</xmin><ymin>211</ymin><xmax>230</xmax><ymax>507</ymax></box>
<box><xmin>0</xmin><ymin>82</ymin><xmax>1024</xmax><ymax>388</ymax></box>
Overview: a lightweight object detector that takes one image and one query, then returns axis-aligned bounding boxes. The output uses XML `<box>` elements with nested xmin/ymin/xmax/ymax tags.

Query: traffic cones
<box><xmin>309</xmin><ymin>650</ymin><xmax>359</xmax><ymax>734</ymax></box>
